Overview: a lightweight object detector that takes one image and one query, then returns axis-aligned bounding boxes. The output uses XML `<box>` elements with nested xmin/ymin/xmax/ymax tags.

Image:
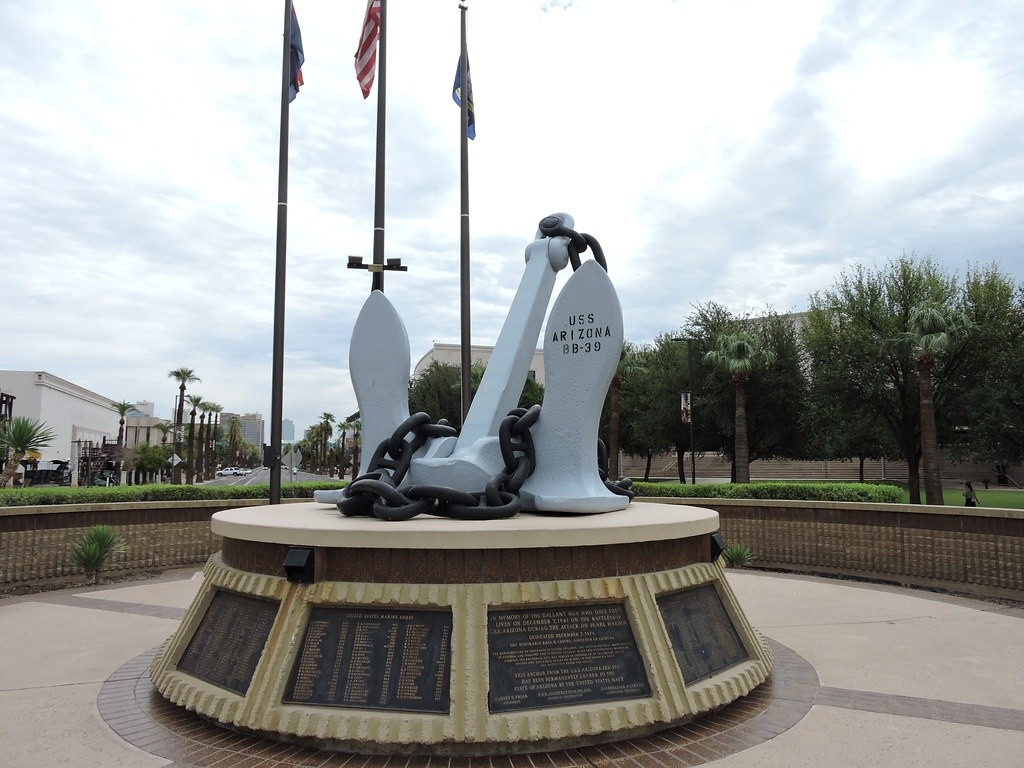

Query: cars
<box><xmin>239</xmin><ymin>467</ymin><xmax>253</xmax><ymax>476</ymax></box>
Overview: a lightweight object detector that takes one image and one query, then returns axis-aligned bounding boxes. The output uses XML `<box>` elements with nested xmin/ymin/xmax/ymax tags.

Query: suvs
<box><xmin>216</xmin><ymin>467</ymin><xmax>240</xmax><ymax>476</ymax></box>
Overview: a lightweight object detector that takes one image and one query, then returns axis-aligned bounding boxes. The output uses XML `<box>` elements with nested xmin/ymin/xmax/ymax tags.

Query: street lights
<box><xmin>670</xmin><ymin>337</ymin><xmax>698</xmax><ymax>484</ymax></box>
<box><xmin>171</xmin><ymin>395</ymin><xmax>192</xmax><ymax>484</ymax></box>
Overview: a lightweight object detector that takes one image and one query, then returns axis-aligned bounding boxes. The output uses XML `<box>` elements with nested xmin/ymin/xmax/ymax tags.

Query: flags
<box><xmin>354</xmin><ymin>1</ymin><xmax>381</xmax><ymax>99</ymax></box>
<box><xmin>451</xmin><ymin>41</ymin><xmax>476</xmax><ymax>140</ymax></box>
<box><xmin>290</xmin><ymin>2</ymin><xmax>305</xmax><ymax>104</ymax></box>
